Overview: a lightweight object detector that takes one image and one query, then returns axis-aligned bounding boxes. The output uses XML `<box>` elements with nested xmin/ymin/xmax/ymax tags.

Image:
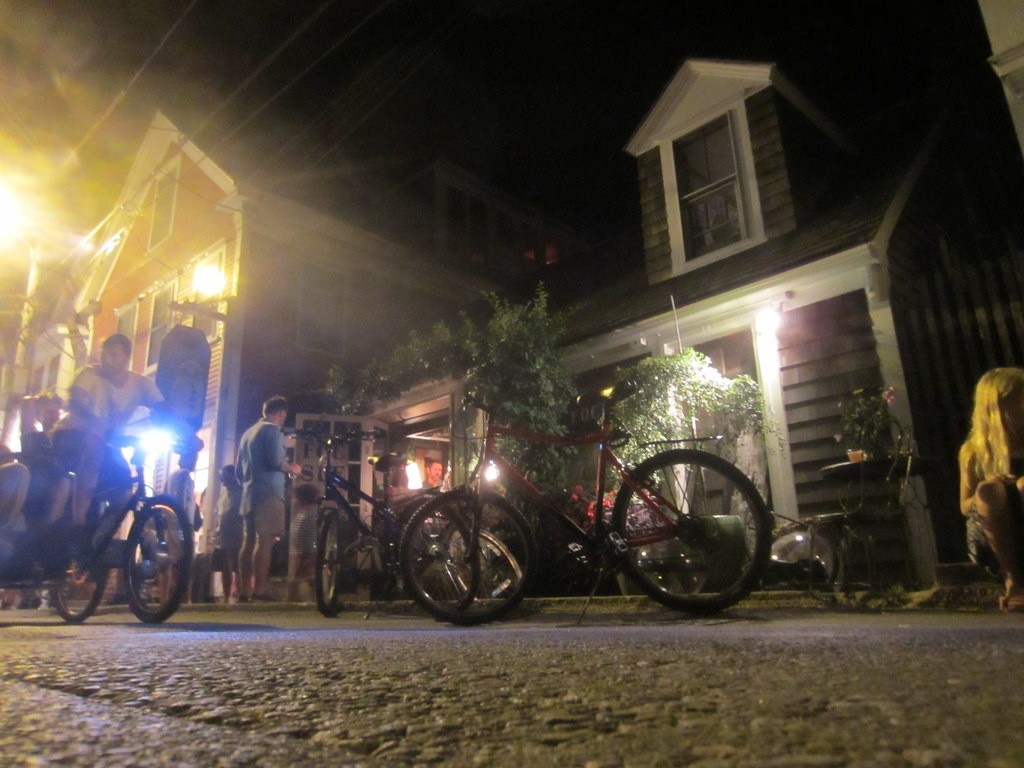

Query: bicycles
<box><xmin>399</xmin><ymin>380</ymin><xmax>775</xmax><ymax>627</ymax></box>
<box><xmin>54</xmin><ymin>433</ymin><xmax>193</xmax><ymax>623</ymax></box>
<box><xmin>281</xmin><ymin>426</ymin><xmax>522</xmax><ymax>619</ymax></box>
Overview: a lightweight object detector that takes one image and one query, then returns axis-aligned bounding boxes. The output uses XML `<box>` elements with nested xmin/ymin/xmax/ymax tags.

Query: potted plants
<box><xmin>601</xmin><ymin>347</ymin><xmax>787</xmax><ymax>596</ymax></box>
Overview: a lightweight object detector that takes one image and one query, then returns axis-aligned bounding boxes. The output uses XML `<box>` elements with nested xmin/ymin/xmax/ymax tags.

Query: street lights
<box><xmin>0</xmin><ymin>206</ymin><xmax>40</xmax><ymax>449</ymax></box>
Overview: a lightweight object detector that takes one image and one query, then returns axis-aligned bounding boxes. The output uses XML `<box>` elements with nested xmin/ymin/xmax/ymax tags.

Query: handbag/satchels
<box><xmin>211</xmin><ymin>544</ymin><xmax>222</xmax><ymax>571</ymax></box>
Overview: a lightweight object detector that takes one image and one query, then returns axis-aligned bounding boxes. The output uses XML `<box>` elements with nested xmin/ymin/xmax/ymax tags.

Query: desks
<box><xmin>818</xmin><ymin>454</ymin><xmax>941</xmax><ymax>583</ymax></box>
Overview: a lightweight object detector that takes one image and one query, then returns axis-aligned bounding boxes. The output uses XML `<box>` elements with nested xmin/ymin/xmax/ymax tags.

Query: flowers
<box><xmin>831</xmin><ymin>383</ymin><xmax>895</xmax><ymax>450</ymax></box>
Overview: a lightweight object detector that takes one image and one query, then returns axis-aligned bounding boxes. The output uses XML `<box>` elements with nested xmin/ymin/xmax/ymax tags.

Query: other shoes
<box><xmin>998</xmin><ymin>593</ymin><xmax>1024</xmax><ymax>613</ymax></box>
<box><xmin>246</xmin><ymin>592</ymin><xmax>274</xmax><ymax>603</ymax></box>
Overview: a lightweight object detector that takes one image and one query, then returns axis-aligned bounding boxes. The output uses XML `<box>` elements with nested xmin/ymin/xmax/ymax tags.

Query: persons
<box><xmin>960</xmin><ymin>367</ymin><xmax>1024</xmax><ymax>611</ymax></box>
<box><xmin>166</xmin><ymin>454</ymin><xmax>195</xmax><ymax>603</ymax></box>
<box><xmin>21</xmin><ymin>390</ymin><xmax>63</xmax><ymax>494</ymax></box>
<box><xmin>191</xmin><ymin>464</ymin><xmax>243</xmax><ymax>602</ymax></box>
<box><xmin>45</xmin><ymin>333</ymin><xmax>203</xmax><ymax>583</ymax></box>
<box><xmin>238</xmin><ymin>396</ymin><xmax>302</xmax><ymax>602</ymax></box>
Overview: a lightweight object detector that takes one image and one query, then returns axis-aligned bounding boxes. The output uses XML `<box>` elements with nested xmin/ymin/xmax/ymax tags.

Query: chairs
<box><xmin>809</xmin><ymin>414</ymin><xmax>916</xmax><ymax>593</ymax></box>
